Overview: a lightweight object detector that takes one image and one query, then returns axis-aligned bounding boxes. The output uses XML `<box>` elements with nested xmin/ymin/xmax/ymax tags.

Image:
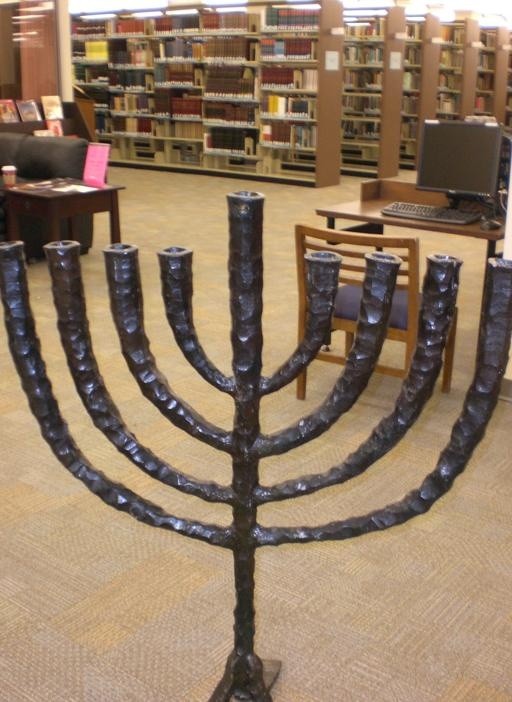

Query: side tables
<box><xmin>4</xmin><ymin>176</ymin><xmax>127</xmax><ymax>246</ymax></box>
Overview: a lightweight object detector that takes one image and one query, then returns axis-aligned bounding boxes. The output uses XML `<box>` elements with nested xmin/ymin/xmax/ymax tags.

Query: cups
<box><xmin>1</xmin><ymin>165</ymin><xmax>17</xmax><ymax>189</ymax></box>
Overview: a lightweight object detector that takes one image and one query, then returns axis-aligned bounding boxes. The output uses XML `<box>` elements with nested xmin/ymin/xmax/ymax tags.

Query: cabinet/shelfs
<box><xmin>77</xmin><ymin>1</ymin><xmax>347</xmax><ymax>186</ymax></box>
<box><xmin>436</xmin><ymin>16</ymin><xmax>477</xmax><ymax>125</ymax></box>
<box><xmin>342</xmin><ymin>4</ymin><xmax>409</xmax><ymax>183</ymax></box>
<box><xmin>472</xmin><ymin>24</ymin><xmax>511</xmax><ymax>132</ymax></box>
<box><xmin>398</xmin><ymin>10</ymin><xmax>440</xmax><ymax>172</ymax></box>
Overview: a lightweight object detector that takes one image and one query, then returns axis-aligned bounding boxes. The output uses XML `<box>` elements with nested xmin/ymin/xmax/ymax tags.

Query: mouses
<box><xmin>480</xmin><ymin>218</ymin><xmax>501</xmax><ymax>230</ymax></box>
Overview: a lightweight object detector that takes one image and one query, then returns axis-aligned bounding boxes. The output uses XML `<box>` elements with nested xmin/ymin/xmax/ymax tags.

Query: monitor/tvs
<box><xmin>414</xmin><ymin>117</ymin><xmax>505</xmax><ymax>209</ymax></box>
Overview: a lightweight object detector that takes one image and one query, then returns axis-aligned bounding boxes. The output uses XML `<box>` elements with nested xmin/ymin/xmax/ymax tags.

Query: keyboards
<box><xmin>381</xmin><ymin>201</ymin><xmax>483</xmax><ymax>226</ymax></box>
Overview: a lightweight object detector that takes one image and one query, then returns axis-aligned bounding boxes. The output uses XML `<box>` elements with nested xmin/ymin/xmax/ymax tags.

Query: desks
<box><xmin>314</xmin><ymin>175</ymin><xmax>505</xmax><ymax>351</ymax></box>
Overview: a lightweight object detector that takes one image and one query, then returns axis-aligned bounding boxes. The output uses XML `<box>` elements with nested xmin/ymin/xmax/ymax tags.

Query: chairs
<box><xmin>0</xmin><ymin>131</ymin><xmax>93</xmax><ymax>263</ymax></box>
<box><xmin>295</xmin><ymin>221</ymin><xmax>456</xmax><ymax>401</ymax></box>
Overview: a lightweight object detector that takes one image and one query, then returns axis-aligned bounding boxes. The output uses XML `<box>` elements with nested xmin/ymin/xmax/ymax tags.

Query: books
<box><xmin>437</xmin><ymin>25</ymin><xmax>465</xmax><ymax>119</ymax></box>
<box><xmin>14</xmin><ymin>142</ymin><xmax>112</xmax><ymax>197</ymax></box>
<box><xmin>70</xmin><ymin>5</ymin><xmax>321</xmax><ymax>162</ymax></box>
<box><xmin>400</xmin><ymin>22</ymin><xmax>425</xmax><ymax>153</ymax></box>
<box><xmin>475</xmin><ymin>30</ymin><xmax>498</xmax><ymax>116</ymax></box>
<box><xmin>1</xmin><ymin>95</ymin><xmax>64</xmax><ymax>123</ymax></box>
<box><xmin>341</xmin><ymin>15</ymin><xmax>381</xmax><ymax>139</ymax></box>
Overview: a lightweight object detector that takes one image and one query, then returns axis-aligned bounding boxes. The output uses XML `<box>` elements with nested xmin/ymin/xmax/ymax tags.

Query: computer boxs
<box><xmin>496</xmin><ymin>133</ymin><xmax>512</xmax><ymax>209</ymax></box>
